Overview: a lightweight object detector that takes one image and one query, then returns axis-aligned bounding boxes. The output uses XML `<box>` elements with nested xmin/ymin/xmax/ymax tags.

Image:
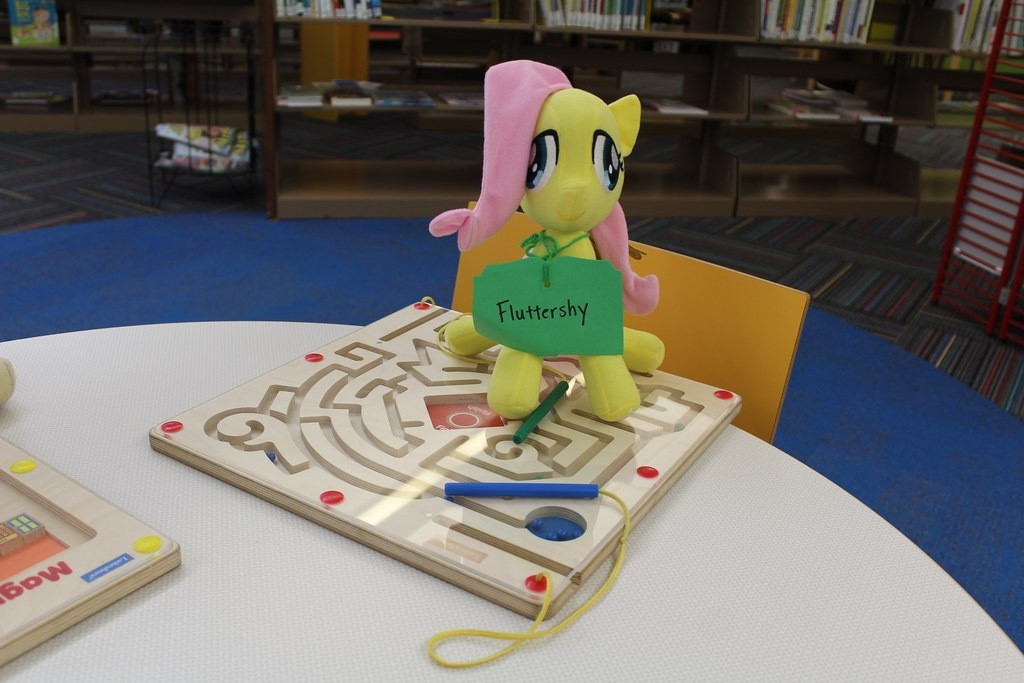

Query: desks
<box><xmin>2</xmin><ymin>320</ymin><xmax>1024</xmax><ymax>682</ymax></box>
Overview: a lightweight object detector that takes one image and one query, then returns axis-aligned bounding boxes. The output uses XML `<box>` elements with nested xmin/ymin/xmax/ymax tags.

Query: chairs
<box><xmin>446</xmin><ymin>195</ymin><xmax>811</xmax><ymax>449</ymax></box>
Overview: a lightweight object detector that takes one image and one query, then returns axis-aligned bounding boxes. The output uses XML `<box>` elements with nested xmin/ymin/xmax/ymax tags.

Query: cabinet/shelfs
<box><xmin>0</xmin><ymin>1</ymin><xmax>1024</xmax><ymax>228</ymax></box>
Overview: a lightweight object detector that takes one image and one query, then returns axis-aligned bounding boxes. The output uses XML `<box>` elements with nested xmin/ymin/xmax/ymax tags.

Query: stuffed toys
<box><xmin>428</xmin><ymin>59</ymin><xmax>666</xmax><ymax>423</ymax></box>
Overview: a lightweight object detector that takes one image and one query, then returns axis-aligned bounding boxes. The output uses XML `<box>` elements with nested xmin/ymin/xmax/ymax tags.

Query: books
<box><xmin>761</xmin><ymin>0</ymin><xmax>875</xmax><ymax>45</ymax></box>
<box><xmin>5</xmin><ymin>91</ymin><xmax>73</xmax><ymax>112</ymax></box>
<box><xmin>439</xmin><ymin>93</ymin><xmax>484</xmax><ymax>105</ymax></box>
<box><xmin>274</xmin><ymin>0</ymin><xmax>382</xmax><ymax>18</ymax></box>
<box><xmin>769</xmin><ymin>102</ymin><xmax>841</xmax><ymax>120</ymax></box>
<box><xmin>99</xmin><ymin>88</ymin><xmax>154</xmax><ymax>106</ymax></box>
<box><xmin>83</xmin><ymin>15</ymin><xmax>129</xmax><ymax>33</ymax></box>
<box><xmin>836</xmin><ymin>105</ymin><xmax>893</xmax><ymax>122</ymax></box>
<box><xmin>276</xmin><ymin>78</ymin><xmax>435</xmax><ymax>107</ymax></box>
<box><xmin>933</xmin><ymin>0</ymin><xmax>1024</xmax><ymax>56</ymax></box>
<box><xmin>642</xmin><ymin>97</ymin><xmax>709</xmax><ymax>114</ymax></box>
<box><xmin>3</xmin><ymin>0</ymin><xmax>59</xmax><ymax>47</ymax></box>
<box><xmin>538</xmin><ymin>0</ymin><xmax>645</xmax><ymax>30</ymax></box>
